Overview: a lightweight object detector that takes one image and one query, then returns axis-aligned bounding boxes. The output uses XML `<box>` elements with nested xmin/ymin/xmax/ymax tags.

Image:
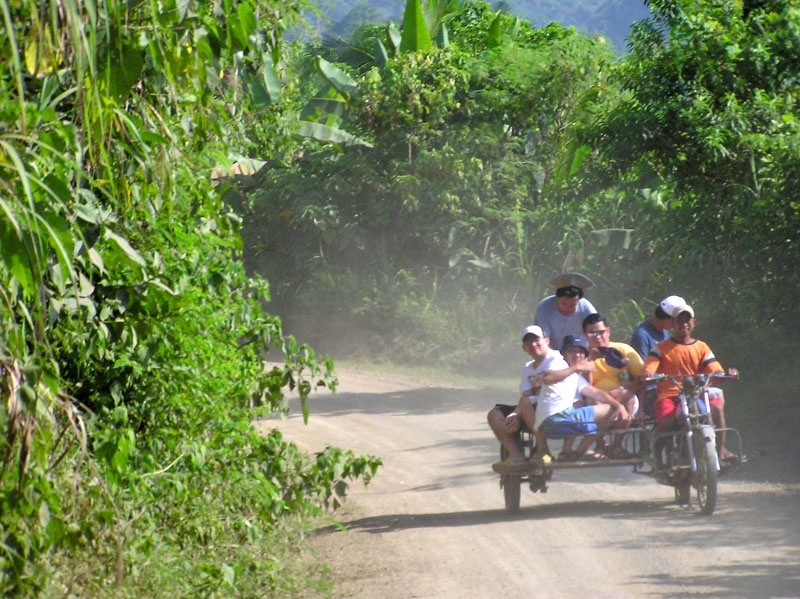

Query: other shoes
<box><xmin>492</xmin><ymin>459</ymin><xmax>529</xmax><ymax>473</ymax></box>
<box><xmin>529</xmin><ymin>453</ymin><xmax>553</xmax><ymax>463</ymax></box>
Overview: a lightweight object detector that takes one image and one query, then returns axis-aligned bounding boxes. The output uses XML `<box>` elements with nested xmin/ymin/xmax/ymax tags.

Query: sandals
<box><xmin>610</xmin><ymin>446</ymin><xmax>629</xmax><ymax>457</ymax></box>
<box><xmin>575</xmin><ymin>448</ymin><xmax>609</xmax><ymax>460</ymax></box>
<box><xmin>557</xmin><ymin>451</ymin><xmax>575</xmax><ymax>462</ymax></box>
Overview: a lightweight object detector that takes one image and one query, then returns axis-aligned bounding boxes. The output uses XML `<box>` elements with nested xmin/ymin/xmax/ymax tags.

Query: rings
<box><xmin>734</xmin><ymin>372</ymin><xmax>737</xmax><ymax>375</ymax></box>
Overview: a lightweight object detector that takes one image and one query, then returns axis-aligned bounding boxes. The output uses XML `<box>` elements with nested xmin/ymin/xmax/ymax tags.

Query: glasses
<box><xmin>587</xmin><ymin>328</ymin><xmax>609</xmax><ymax>338</ymax></box>
<box><xmin>556</xmin><ymin>291</ymin><xmax>576</xmax><ymax>297</ymax></box>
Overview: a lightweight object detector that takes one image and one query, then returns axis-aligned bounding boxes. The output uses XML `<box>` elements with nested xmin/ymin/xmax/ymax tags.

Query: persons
<box><xmin>532</xmin><ymin>334</ymin><xmax>631</xmax><ymax>463</ymax></box>
<box><xmin>641</xmin><ymin>304</ymin><xmax>748</xmax><ymax>467</ymax></box>
<box><xmin>533</xmin><ymin>271</ymin><xmax>599</xmax><ymax>351</ymax></box>
<box><xmin>573</xmin><ymin>314</ymin><xmax>647</xmax><ymax>461</ymax></box>
<box><xmin>629</xmin><ymin>294</ymin><xmax>687</xmax><ymax>363</ymax></box>
<box><xmin>488</xmin><ymin>326</ymin><xmax>564</xmax><ymax>475</ymax></box>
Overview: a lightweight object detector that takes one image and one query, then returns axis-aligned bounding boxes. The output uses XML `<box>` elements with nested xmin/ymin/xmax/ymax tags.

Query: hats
<box><xmin>560</xmin><ymin>335</ymin><xmax>590</xmax><ymax>358</ymax></box>
<box><xmin>671</xmin><ymin>306</ymin><xmax>695</xmax><ymax>319</ymax></box>
<box><xmin>521</xmin><ymin>326</ymin><xmax>547</xmax><ymax>341</ymax></box>
<box><xmin>660</xmin><ymin>295</ymin><xmax>686</xmax><ymax>317</ymax></box>
<box><xmin>598</xmin><ymin>347</ymin><xmax>630</xmax><ymax>368</ymax></box>
<box><xmin>551</xmin><ymin>272</ymin><xmax>594</xmax><ymax>292</ymax></box>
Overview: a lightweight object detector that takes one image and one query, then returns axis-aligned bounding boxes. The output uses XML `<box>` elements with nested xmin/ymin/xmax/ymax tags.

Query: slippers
<box><xmin>719</xmin><ymin>454</ymin><xmax>749</xmax><ymax>465</ymax></box>
<box><xmin>653</xmin><ymin>465</ymin><xmax>672</xmax><ymax>481</ymax></box>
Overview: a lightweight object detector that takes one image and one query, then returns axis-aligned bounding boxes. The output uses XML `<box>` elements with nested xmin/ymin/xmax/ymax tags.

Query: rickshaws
<box><xmin>499</xmin><ymin>371</ymin><xmax>748</xmax><ymax>515</ymax></box>
